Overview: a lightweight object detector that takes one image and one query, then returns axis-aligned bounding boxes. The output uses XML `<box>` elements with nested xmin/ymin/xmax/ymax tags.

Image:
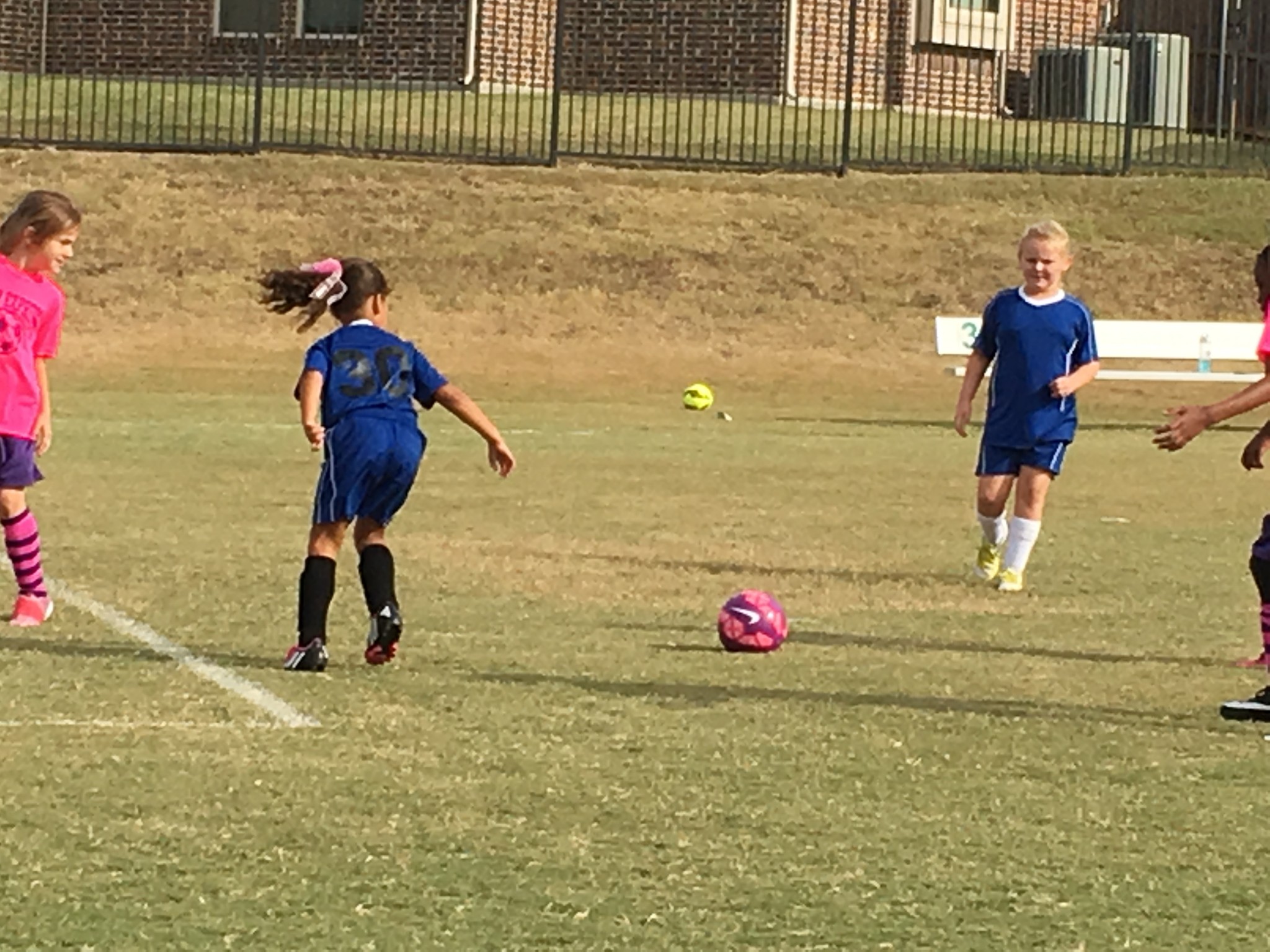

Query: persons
<box><xmin>0</xmin><ymin>191</ymin><xmax>83</xmax><ymax>628</ymax></box>
<box><xmin>954</xmin><ymin>220</ymin><xmax>1100</xmax><ymax>591</ymax></box>
<box><xmin>254</xmin><ymin>256</ymin><xmax>516</xmax><ymax>672</ymax></box>
<box><xmin>1152</xmin><ymin>245</ymin><xmax>1270</xmax><ymax>721</ymax></box>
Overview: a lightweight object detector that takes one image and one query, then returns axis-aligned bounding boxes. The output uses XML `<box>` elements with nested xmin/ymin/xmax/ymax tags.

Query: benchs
<box><xmin>932</xmin><ymin>312</ymin><xmax>1265</xmax><ymax>410</ymax></box>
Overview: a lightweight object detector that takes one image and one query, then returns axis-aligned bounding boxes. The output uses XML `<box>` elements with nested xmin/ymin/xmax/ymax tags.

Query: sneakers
<box><xmin>363</xmin><ymin>600</ymin><xmax>403</xmax><ymax>667</ymax></box>
<box><xmin>998</xmin><ymin>566</ymin><xmax>1024</xmax><ymax>592</ymax></box>
<box><xmin>975</xmin><ymin>519</ymin><xmax>1008</xmax><ymax>580</ymax></box>
<box><xmin>1219</xmin><ymin>684</ymin><xmax>1270</xmax><ymax>724</ymax></box>
<box><xmin>8</xmin><ymin>594</ymin><xmax>54</xmax><ymax>627</ymax></box>
<box><xmin>283</xmin><ymin>637</ymin><xmax>329</xmax><ymax>673</ymax></box>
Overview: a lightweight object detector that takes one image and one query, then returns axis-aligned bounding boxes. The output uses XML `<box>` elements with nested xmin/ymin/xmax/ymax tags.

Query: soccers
<box><xmin>716</xmin><ymin>589</ymin><xmax>788</xmax><ymax>653</ymax></box>
<box><xmin>683</xmin><ymin>382</ymin><xmax>715</xmax><ymax>413</ymax></box>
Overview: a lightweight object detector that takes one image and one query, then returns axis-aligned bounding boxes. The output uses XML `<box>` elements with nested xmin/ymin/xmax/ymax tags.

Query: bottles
<box><xmin>1196</xmin><ymin>336</ymin><xmax>1212</xmax><ymax>374</ymax></box>
